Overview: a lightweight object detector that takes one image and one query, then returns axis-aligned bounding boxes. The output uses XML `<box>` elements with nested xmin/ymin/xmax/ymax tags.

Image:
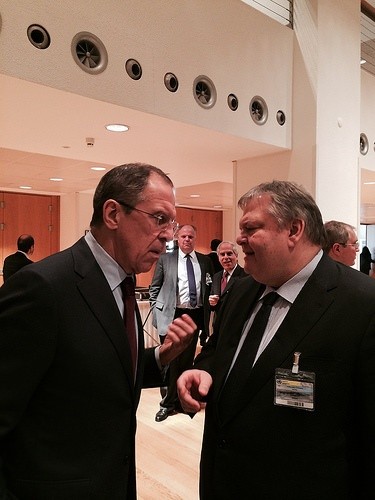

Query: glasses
<box><xmin>117</xmin><ymin>200</ymin><xmax>179</xmax><ymax>235</ymax></box>
<box><xmin>345</xmin><ymin>243</ymin><xmax>359</xmax><ymax>249</ymax></box>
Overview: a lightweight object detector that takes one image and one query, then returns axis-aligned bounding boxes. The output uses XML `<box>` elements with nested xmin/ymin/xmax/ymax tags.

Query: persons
<box><xmin>0</xmin><ymin>163</ymin><xmax>197</xmax><ymax>500</ymax></box>
<box><xmin>177</xmin><ymin>180</ymin><xmax>375</xmax><ymax>500</ymax></box>
<box><xmin>149</xmin><ymin>221</ymin><xmax>375</xmax><ymax>421</ymax></box>
<box><xmin>3</xmin><ymin>234</ymin><xmax>35</xmax><ymax>283</ymax></box>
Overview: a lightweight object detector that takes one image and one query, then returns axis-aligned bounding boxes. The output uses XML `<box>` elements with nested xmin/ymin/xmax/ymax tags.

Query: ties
<box><xmin>186</xmin><ymin>255</ymin><xmax>199</xmax><ymax>308</ymax></box>
<box><xmin>220</xmin><ymin>272</ymin><xmax>230</xmax><ymax>312</ymax></box>
<box><xmin>120</xmin><ymin>277</ymin><xmax>137</xmax><ymax>385</ymax></box>
<box><xmin>212</xmin><ymin>292</ymin><xmax>279</xmax><ymax>450</ymax></box>
